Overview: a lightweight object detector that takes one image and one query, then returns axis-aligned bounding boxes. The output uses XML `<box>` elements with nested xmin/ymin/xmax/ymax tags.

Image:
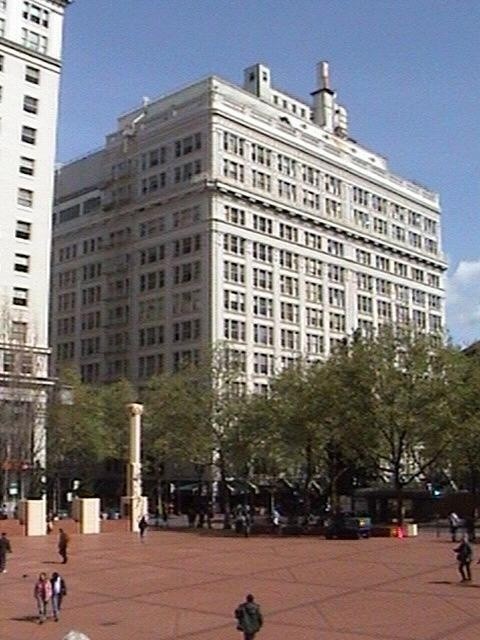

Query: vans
<box><xmin>323</xmin><ymin>509</ymin><xmax>372</xmax><ymax>540</ymax></box>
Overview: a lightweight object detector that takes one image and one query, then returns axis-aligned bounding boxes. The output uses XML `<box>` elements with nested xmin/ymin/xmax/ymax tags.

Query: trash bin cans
<box><xmin>407</xmin><ymin>521</ymin><xmax>418</xmax><ymax>536</ymax></box>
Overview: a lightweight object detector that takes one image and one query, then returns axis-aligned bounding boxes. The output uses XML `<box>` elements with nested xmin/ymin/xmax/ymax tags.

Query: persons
<box><xmin>449</xmin><ymin>509</ymin><xmax>463</xmax><ymax>541</ymax></box>
<box><xmin>0</xmin><ymin>532</ymin><xmax>14</xmax><ymax>573</ymax></box>
<box><xmin>57</xmin><ymin>528</ymin><xmax>70</xmax><ymax>564</ymax></box>
<box><xmin>33</xmin><ymin>571</ymin><xmax>53</xmax><ymax>624</ymax></box>
<box><xmin>138</xmin><ymin>515</ymin><xmax>150</xmax><ymax>541</ymax></box>
<box><xmin>450</xmin><ymin>533</ymin><xmax>474</xmax><ymax>583</ymax></box>
<box><xmin>48</xmin><ymin>572</ymin><xmax>67</xmax><ymax>622</ymax></box>
<box><xmin>243</xmin><ymin>509</ymin><xmax>252</xmax><ymax>538</ymax></box>
<box><xmin>235</xmin><ymin>593</ymin><xmax>264</xmax><ymax>640</ymax></box>
